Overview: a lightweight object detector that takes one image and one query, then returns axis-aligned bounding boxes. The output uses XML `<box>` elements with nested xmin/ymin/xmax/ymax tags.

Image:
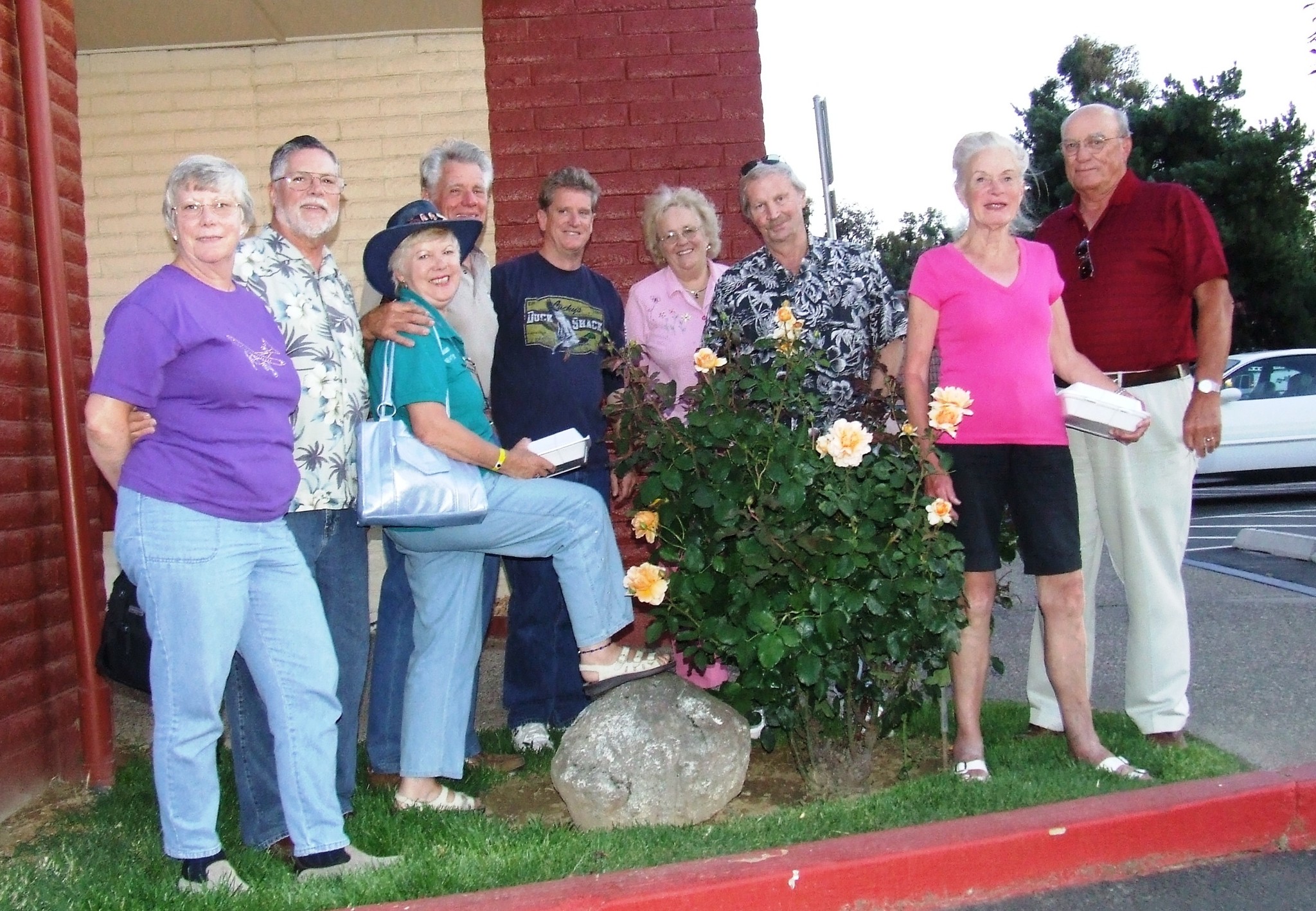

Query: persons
<box><xmin>902</xmin><ymin>133</ymin><xmax>1154</xmax><ymax>788</ymax></box>
<box><xmin>84</xmin><ymin>156</ymin><xmax>403</xmax><ymax>895</ymax></box>
<box><xmin>129</xmin><ymin>134</ymin><xmax>371</xmax><ymax>859</ymax></box>
<box><xmin>1024</xmin><ymin>103</ymin><xmax>1233</xmax><ymax>749</ymax></box>
<box><xmin>356</xmin><ymin>137</ymin><xmax>910</xmax><ymax>808</ymax></box>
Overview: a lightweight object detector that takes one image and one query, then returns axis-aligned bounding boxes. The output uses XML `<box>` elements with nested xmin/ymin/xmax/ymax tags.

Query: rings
<box><xmin>1205</xmin><ymin>436</ymin><xmax>1214</xmax><ymax>441</ymax></box>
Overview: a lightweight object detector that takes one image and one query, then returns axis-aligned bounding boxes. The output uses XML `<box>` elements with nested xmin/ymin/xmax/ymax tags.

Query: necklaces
<box><xmin>684</xmin><ymin>286</ymin><xmax>707</xmax><ymax>298</ymax></box>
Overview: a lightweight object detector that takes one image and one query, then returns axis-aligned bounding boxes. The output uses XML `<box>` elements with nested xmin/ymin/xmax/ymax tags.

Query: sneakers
<box><xmin>511</xmin><ymin>722</ymin><xmax>553</xmax><ymax>754</ymax></box>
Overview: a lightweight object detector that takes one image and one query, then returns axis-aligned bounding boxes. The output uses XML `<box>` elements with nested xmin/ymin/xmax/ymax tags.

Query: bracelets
<box><xmin>1114</xmin><ymin>387</ymin><xmax>1127</xmax><ymax>395</ymax></box>
<box><xmin>492</xmin><ymin>448</ymin><xmax>506</xmax><ymax>471</ymax></box>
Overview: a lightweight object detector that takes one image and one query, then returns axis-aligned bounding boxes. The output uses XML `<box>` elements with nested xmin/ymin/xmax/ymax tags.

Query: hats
<box><xmin>363</xmin><ymin>199</ymin><xmax>484</xmax><ymax>299</ymax></box>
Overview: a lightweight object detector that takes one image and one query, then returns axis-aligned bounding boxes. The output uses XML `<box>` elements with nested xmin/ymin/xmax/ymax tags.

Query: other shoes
<box><xmin>177</xmin><ymin>861</ymin><xmax>252</xmax><ymax>894</ymax></box>
<box><xmin>297</xmin><ymin>843</ymin><xmax>404</xmax><ymax>885</ymax></box>
<box><xmin>1018</xmin><ymin>723</ymin><xmax>1062</xmax><ymax>743</ymax></box>
<box><xmin>271</xmin><ymin>837</ymin><xmax>294</xmax><ymax>864</ymax></box>
<box><xmin>1147</xmin><ymin>731</ymin><xmax>1190</xmax><ymax>751</ymax></box>
<box><xmin>467</xmin><ymin>750</ymin><xmax>525</xmax><ymax>774</ymax></box>
<box><xmin>368</xmin><ymin>770</ymin><xmax>401</xmax><ymax>791</ymax></box>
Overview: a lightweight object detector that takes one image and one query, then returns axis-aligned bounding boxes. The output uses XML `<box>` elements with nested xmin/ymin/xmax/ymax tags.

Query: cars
<box><xmin>1191</xmin><ymin>347</ymin><xmax>1316</xmax><ymax>501</ymax></box>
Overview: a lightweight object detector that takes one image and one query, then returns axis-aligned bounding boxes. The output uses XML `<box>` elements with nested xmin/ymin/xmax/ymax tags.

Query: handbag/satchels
<box><xmin>97</xmin><ymin>568</ymin><xmax>152</xmax><ymax>695</ymax></box>
<box><xmin>355</xmin><ymin>321</ymin><xmax>487</xmax><ymax>527</ymax></box>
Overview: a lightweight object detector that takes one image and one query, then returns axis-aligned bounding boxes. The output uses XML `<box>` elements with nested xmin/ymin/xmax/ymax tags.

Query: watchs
<box><xmin>1192</xmin><ymin>378</ymin><xmax>1222</xmax><ymax>394</ymax></box>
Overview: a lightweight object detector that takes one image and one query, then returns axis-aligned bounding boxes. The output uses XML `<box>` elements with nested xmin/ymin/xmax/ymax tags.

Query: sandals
<box><xmin>1095</xmin><ymin>754</ymin><xmax>1151</xmax><ymax>779</ymax></box>
<box><xmin>391</xmin><ymin>785</ymin><xmax>485</xmax><ymax>819</ymax></box>
<box><xmin>578</xmin><ymin>645</ymin><xmax>676</xmax><ymax>695</ymax></box>
<box><xmin>955</xmin><ymin>759</ymin><xmax>991</xmax><ymax>782</ymax></box>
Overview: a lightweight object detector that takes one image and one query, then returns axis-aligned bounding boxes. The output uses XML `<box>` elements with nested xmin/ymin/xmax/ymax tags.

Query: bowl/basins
<box><xmin>529</xmin><ymin>427</ymin><xmax>592</xmax><ymax>481</ymax></box>
<box><xmin>1057</xmin><ymin>382</ymin><xmax>1151</xmax><ymax>440</ymax></box>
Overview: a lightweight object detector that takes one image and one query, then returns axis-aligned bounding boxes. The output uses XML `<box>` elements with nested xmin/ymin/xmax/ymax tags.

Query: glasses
<box><xmin>661</xmin><ymin>227</ymin><xmax>699</xmax><ymax>245</ymax></box>
<box><xmin>741</xmin><ymin>155</ymin><xmax>780</xmax><ymax>176</ymax></box>
<box><xmin>275</xmin><ymin>171</ymin><xmax>347</xmax><ymax>194</ymax></box>
<box><xmin>1074</xmin><ymin>239</ymin><xmax>1094</xmax><ymax>280</ymax></box>
<box><xmin>170</xmin><ymin>200</ymin><xmax>241</xmax><ymax>218</ymax></box>
<box><xmin>1058</xmin><ymin>136</ymin><xmax>1118</xmax><ymax>156</ymax></box>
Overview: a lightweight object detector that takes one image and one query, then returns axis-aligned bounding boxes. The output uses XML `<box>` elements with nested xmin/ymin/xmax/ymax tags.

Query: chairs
<box><xmin>1282</xmin><ymin>373</ymin><xmax>1313</xmax><ymax>397</ymax></box>
<box><xmin>1247</xmin><ymin>382</ymin><xmax>1275</xmax><ymax>399</ymax></box>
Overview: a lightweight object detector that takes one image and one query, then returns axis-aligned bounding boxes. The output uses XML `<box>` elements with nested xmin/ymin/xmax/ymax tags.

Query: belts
<box><xmin>1056</xmin><ymin>362</ymin><xmax>1193</xmax><ymax>390</ymax></box>
